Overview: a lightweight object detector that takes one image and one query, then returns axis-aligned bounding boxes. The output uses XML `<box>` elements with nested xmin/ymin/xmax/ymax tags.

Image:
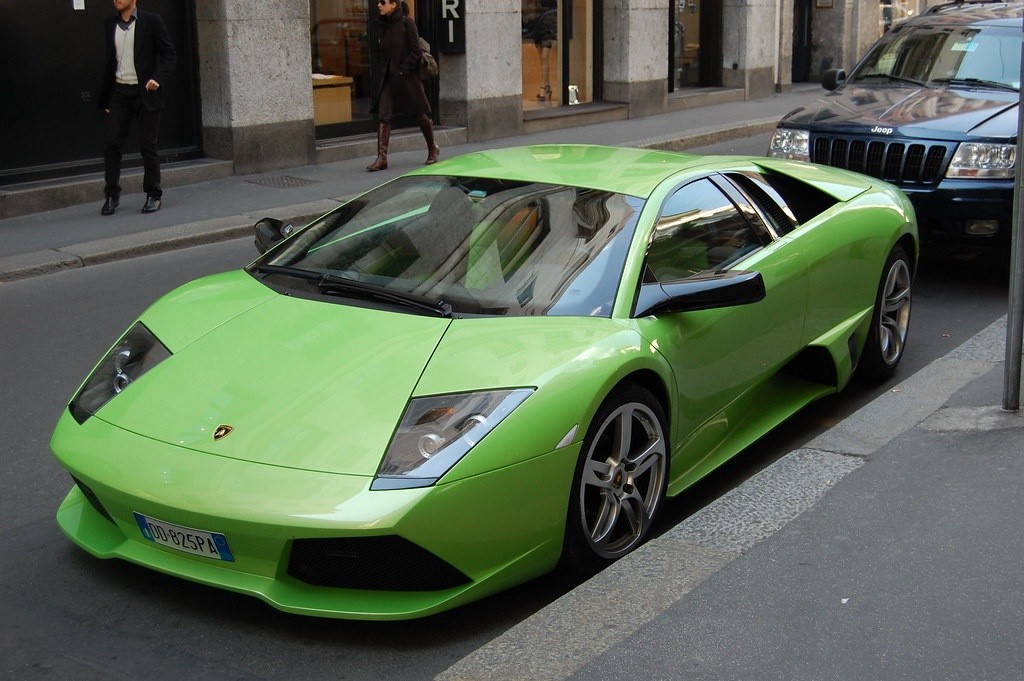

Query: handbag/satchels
<box><xmin>404</xmin><ymin>16</ymin><xmax>439</xmax><ymax>80</ymax></box>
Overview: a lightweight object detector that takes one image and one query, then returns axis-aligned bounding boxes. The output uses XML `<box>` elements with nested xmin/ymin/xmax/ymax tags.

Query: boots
<box><xmin>417</xmin><ymin>116</ymin><xmax>439</xmax><ymax>164</ymax></box>
<box><xmin>366</xmin><ymin>122</ymin><xmax>391</xmax><ymax>170</ymax></box>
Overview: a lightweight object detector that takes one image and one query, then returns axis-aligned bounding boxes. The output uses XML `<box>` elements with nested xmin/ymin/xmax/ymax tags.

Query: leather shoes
<box><xmin>102</xmin><ymin>196</ymin><xmax>119</xmax><ymax>215</ymax></box>
<box><xmin>142</xmin><ymin>197</ymin><xmax>161</xmax><ymax>213</ymax></box>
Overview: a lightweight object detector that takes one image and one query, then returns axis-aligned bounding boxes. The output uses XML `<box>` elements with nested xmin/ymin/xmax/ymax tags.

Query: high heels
<box><xmin>538</xmin><ymin>87</ymin><xmax>546</xmax><ymax>101</ymax></box>
<box><xmin>537</xmin><ymin>86</ymin><xmax>552</xmax><ymax>98</ymax></box>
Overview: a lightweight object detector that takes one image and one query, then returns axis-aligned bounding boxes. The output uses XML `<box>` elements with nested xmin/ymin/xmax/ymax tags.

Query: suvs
<box><xmin>766</xmin><ymin>0</ymin><xmax>1024</xmax><ymax>287</ymax></box>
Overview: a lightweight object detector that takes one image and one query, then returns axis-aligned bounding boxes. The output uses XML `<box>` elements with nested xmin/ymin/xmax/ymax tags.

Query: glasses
<box><xmin>376</xmin><ymin>0</ymin><xmax>389</xmax><ymax>5</ymax></box>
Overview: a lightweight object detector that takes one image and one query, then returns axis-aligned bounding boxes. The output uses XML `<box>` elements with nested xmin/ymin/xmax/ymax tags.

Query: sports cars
<box><xmin>50</xmin><ymin>142</ymin><xmax>924</xmax><ymax>620</ymax></box>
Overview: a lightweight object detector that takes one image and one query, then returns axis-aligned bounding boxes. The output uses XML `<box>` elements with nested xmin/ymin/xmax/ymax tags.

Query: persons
<box><xmin>366</xmin><ymin>0</ymin><xmax>440</xmax><ymax>171</ymax></box>
<box><xmin>102</xmin><ymin>0</ymin><xmax>179</xmax><ymax>215</ymax></box>
<box><xmin>526</xmin><ymin>0</ymin><xmax>557</xmax><ymax>101</ymax></box>
